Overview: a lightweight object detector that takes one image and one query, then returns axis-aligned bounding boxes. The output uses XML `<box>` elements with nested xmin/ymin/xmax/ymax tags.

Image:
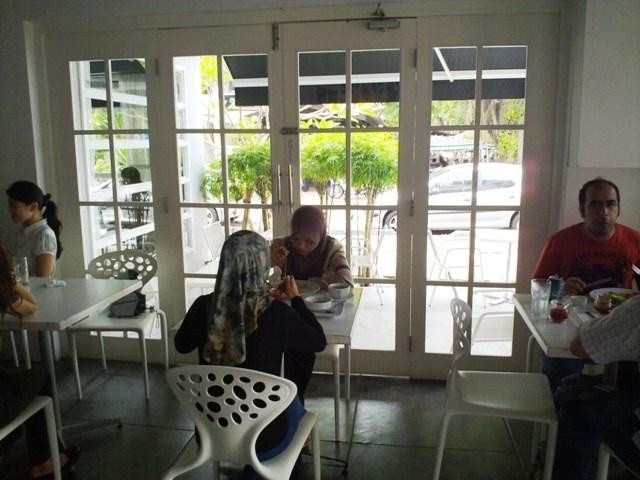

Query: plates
<box><xmin>271</xmin><ymin>279</ymin><xmax>320</xmax><ymax>297</ymax></box>
<box><xmin>313</xmin><ymin>300</ymin><xmax>347</xmax><ymax>317</ymax></box>
<box><xmin>589</xmin><ymin>288</ymin><xmax>631</xmax><ymax>299</ymax></box>
<box><xmin>326</xmin><ymin>292</ymin><xmax>354</xmax><ymax>300</ymax></box>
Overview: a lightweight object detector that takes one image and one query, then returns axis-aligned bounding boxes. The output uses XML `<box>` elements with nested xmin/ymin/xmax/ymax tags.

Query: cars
<box><xmin>198</xmin><ymin>199</ymin><xmax>240</xmax><ymax>222</ymax></box>
<box><xmin>374</xmin><ymin>161</ymin><xmax>522</xmax><ymax>230</ymax></box>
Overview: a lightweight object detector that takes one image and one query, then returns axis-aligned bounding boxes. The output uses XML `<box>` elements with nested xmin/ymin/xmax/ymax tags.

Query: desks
<box><xmin>482</xmin><ymin>230</ymin><xmax>517</xmax><ymax>306</ymax></box>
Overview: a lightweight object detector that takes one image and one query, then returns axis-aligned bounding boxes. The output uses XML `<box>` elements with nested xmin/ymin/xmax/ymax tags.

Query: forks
<box><xmin>632</xmin><ymin>264</ymin><xmax>640</xmax><ymax>276</ymax></box>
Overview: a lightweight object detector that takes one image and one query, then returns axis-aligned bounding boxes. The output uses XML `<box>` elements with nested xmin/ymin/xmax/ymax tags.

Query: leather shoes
<box><xmin>31</xmin><ymin>444</ymin><xmax>81</xmax><ymax>479</ymax></box>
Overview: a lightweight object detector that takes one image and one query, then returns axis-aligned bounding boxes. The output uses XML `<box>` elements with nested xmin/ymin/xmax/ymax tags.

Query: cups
<box><xmin>531</xmin><ymin>278</ymin><xmax>552</xmax><ymax>316</ymax></box>
<box><xmin>12</xmin><ymin>256</ymin><xmax>28</xmax><ymax>285</ymax></box>
<box><xmin>550</xmin><ymin>298</ymin><xmax>567</xmax><ymax>323</ymax></box>
<box><xmin>593</xmin><ymin>293</ymin><xmax>612</xmax><ymax>314</ymax></box>
<box><xmin>571</xmin><ymin>296</ymin><xmax>587</xmax><ymax>305</ymax></box>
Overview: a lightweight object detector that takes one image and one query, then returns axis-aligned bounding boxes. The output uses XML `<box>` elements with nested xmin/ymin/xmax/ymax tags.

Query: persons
<box><xmin>175</xmin><ymin>231</ymin><xmax>328</xmax><ymax>480</ymax></box>
<box><xmin>6</xmin><ymin>181</ymin><xmax>62</xmax><ymax>278</ymax></box>
<box><xmin>109</xmin><ymin>167</ymin><xmax>149</xmax><ymax>228</ymax></box>
<box><xmin>532</xmin><ymin>179</ymin><xmax>640</xmax><ymax>449</ymax></box>
<box><xmin>0</xmin><ymin>245</ymin><xmax>80</xmax><ymax>480</ymax></box>
<box><xmin>570</xmin><ymin>295</ymin><xmax>640</xmax><ymax>471</ymax></box>
<box><xmin>270</xmin><ymin>206</ymin><xmax>354</xmax><ymax>290</ymax></box>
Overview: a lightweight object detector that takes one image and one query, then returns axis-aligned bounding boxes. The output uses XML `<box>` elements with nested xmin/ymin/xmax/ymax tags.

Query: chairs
<box><xmin>68</xmin><ymin>250</ymin><xmax>168</xmax><ymax>400</ymax></box>
<box><xmin>433</xmin><ymin>299</ymin><xmax>557</xmax><ymax>479</ymax></box>
<box><xmin>204</xmin><ymin>221</ymin><xmax>225</xmax><ymax>257</ymax></box>
<box><xmin>312</xmin><ymin>341</ymin><xmax>342</xmax><ymax>441</ymax></box>
<box><xmin>182</xmin><ymin>247</ymin><xmax>214</xmax><ymax>296</ymax></box>
<box><xmin>597</xmin><ymin>442</ymin><xmax>638</xmax><ymax>479</ymax></box>
<box><xmin>426</xmin><ymin>229</ymin><xmax>484</xmax><ymax>307</ymax></box>
<box><xmin>163</xmin><ymin>365</ymin><xmax>321</xmax><ymax>479</ymax></box>
<box><xmin>339</xmin><ymin>226</ymin><xmax>387</xmax><ymax>307</ymax></box>
<box><xmin>1</xmin><ymin>395</ymin><xmax>62</xmax><ymax>480</ymax></box>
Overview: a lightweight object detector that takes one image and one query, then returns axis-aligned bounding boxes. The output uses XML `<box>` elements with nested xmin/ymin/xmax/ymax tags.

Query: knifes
<box><xmin>586</xmin><ymin>276</ymin><xmax>611</xmax><ymax>287</ymax></box>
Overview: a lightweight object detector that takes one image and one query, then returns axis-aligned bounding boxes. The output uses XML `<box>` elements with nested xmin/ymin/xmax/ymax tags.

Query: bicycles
<box><xmin>327</xmin><ymin>182</ymin><xmax>344</xmax><ymax>198</ymax></box>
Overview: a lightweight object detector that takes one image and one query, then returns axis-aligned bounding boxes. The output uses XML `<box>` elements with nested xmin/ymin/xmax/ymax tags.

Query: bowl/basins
<box><xmin>328</xmin><ymin>283</ymin><xmax>350</xmax><ymax>298</ymax></box>
<box><xmin>304</xmin><ymin>295</ymin><xmax>334</xmax><ymax>312</ymax></box>
